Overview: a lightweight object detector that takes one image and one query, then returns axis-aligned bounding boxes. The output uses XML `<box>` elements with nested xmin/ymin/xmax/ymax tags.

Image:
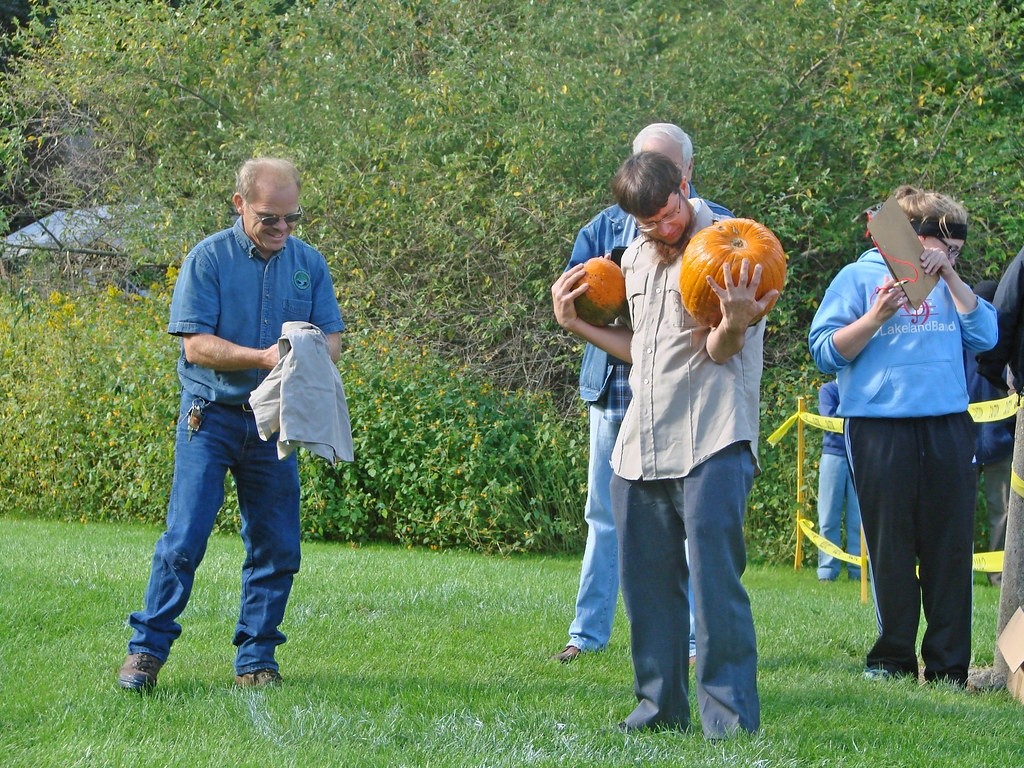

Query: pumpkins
<box><xmin>678</xmin><ymin>218</ymin><xmax>786</xmax><ymax>329</ymax></box>
<box><xmin>569</xmin><ymin>257</ymin><xmax>626</xmax><ymax>327</ymax></box>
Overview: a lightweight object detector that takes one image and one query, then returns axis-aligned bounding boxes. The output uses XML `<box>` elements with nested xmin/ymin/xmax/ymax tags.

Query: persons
<box><xmin>551</xmin><ymin>123</ymin><xmax>780</xmax><ymax>745</ymax></box>
<box><xmin>116</xmin><ymin>156</ymin><xmax>345</xmax><ymax>695</ymax></box>
<box><xmin>809</xmin><ymin>186</ymin><xmax>1024</xmax><ymax>692</ymax></box>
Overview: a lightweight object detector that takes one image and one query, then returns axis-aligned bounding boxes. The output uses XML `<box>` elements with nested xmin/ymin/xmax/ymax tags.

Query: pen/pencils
<box><xmin>876</xmin><ymin>280</ymin><xmax>908</xmax><ymax>294</ymax></box>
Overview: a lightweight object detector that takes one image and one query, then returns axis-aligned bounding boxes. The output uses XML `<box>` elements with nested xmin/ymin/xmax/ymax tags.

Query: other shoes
<box><xmin>552</xmin><ymin>646</ymin><xmax>580</xmax><ymax>664</ymax></box>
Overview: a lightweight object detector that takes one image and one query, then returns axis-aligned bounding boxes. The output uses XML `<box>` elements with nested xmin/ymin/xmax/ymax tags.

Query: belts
<box><xmin>243</xmin><ymin>404</ymin><xmax>253</xmax><ymax>412</ymax></box>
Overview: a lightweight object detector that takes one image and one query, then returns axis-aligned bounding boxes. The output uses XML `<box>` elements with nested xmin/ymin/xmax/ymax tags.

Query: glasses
<box><xmin>633</xmin><ymin>192</ymin><xmax>682</xmax><ymax>232</ymax></box>
<box><xmin>249</xmin><ymin>204</ymin><xmax>302</xmax><ymax>226</ymax></box>
<box><xmin>937</xmin><ymin>236</ymin><xmax>960</xmax><ymax>258</ymax></box>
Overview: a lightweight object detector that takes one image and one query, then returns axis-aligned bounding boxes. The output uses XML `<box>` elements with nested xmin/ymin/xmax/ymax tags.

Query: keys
<box><xmin>180</xmin><ymin>398</ymin><xmax>207</xmax><ymax>443</ymax></box>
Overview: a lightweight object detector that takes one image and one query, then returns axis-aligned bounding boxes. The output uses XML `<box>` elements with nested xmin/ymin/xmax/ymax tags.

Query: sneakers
<box><xmin>119</xmin><ymin>653</ymin><xmax>164</xmax><ymax>694</ymax></box>
<box><xmin>235</xmin><ymin>670</ymin><xmax>285</xmax><ymax>688</ymax></box>
